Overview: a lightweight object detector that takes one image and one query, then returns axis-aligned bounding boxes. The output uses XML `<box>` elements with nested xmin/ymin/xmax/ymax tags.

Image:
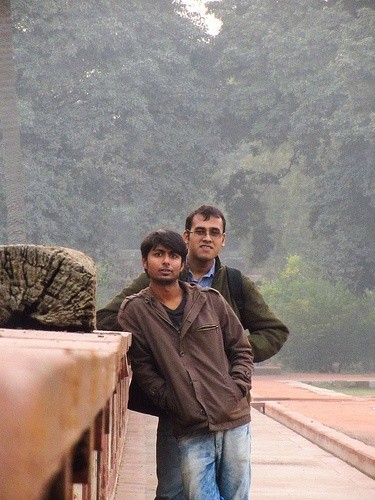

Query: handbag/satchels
<box><xmin>126</xmin><ymin>375</ymin><xmax>161</xmax><ymax>419</ymax></box>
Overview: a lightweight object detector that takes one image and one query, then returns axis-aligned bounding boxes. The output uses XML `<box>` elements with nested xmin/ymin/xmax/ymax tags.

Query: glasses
<box><xmin>186</xmin><ymin>227</ymin><xmax>224</xmax><ymax>238</ymax></box>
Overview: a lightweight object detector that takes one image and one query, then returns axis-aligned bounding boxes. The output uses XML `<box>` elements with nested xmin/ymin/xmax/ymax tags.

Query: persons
<box><xmin>116</xmin><ymin>227</ymin><xmax>255</xmax><ymax>500</ymax></box>
<box><xmin>95</xmin><ymin>204</ymin><xmax>291</xmax><ymax>500</ymax></box>
